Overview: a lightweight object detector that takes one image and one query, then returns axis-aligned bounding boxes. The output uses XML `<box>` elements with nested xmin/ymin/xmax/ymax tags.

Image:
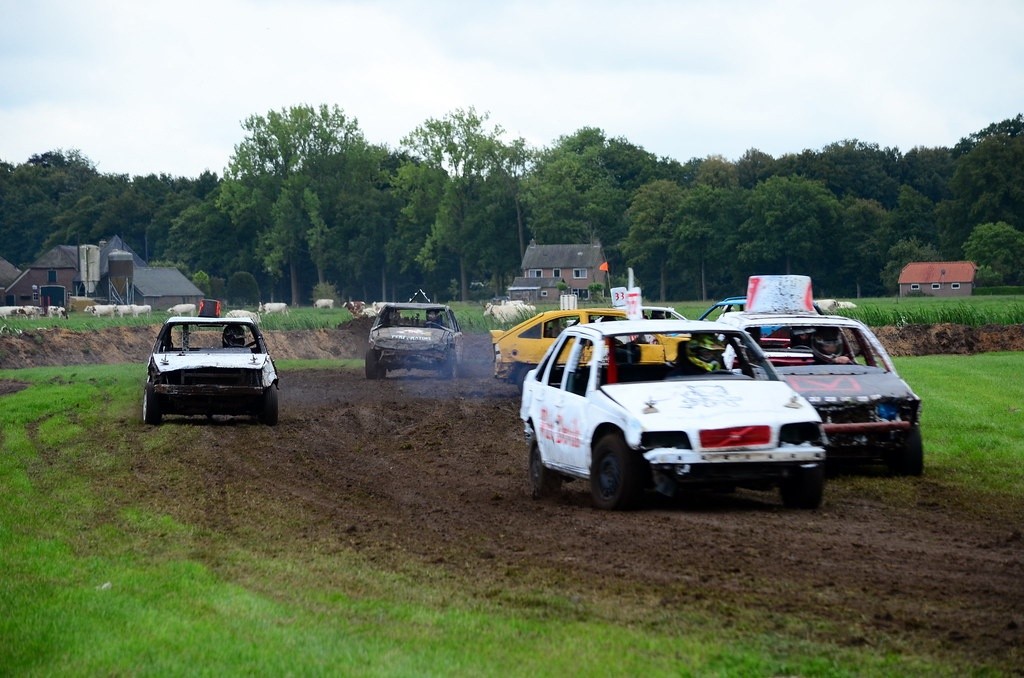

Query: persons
<box><xmin>220</xmin><ymin>326</ymin><xmax>246</xmax><ymax>348</ymax></box>
<box><xmin>807</xmin><ymin>327</ymin><xmax>849</xmax><ymax>364</ymax></box>
<box><xmin>676</xmin><ymin>334</ymin><xmax>726</xmax><ymax>378</ymax></box>
<box><xmin>424</xmin><ymin>310</ymin><xmax>444</xmax><ymax>328</ymax></box>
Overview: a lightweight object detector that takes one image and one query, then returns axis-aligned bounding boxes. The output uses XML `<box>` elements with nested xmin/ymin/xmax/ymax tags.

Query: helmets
<box><xmin>685</xmin><ymin>333</ymin><xmax>727</xmax><ymax>374</ymax></box>
<box><xmin>811</xmin><ymin>328</ymin><xmax>843</xmax><ymax>363</ymax></box>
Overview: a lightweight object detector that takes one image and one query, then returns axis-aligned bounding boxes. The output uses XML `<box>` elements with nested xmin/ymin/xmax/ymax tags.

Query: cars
<box><xmin>607</xmin><ymin>303</ymin><xmax>693</xmax><ymax>320</ymax></box>
<box><xmin>365</xmin><ymin>301</ymin><xmax>464</xmax><ymax>381</ymax></box>
<box><xmin>519</xmin><ymin>320</ymin><xmax>832</xmax><ymax>509</ymax></box>
<box><xmin>141</xmin><ymin>316</ymin><xmax>279</xmax><ymax>426</ymax></box>
<box><xmin>716</xmin><ymin>310</ymin><xmax>925</xmax><ymax>477</ymax></box>
<box><xmin>487</xmin><ymin>307</ymin><xmax>691</xmax><ymax>392</ymax></box>
<box><xmin>698</xmin><ymin>296</ymin><xmax>826</xmax><ymax>327</ymax></box>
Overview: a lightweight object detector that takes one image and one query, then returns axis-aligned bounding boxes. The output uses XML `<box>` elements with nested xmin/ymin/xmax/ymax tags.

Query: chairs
<box><xmin>678</xmin><ymin>340</ymin><xmax>689</xmax><ymax>360</ymax></box>
<box><xmin>386</xmin><ymin>311</ymin><xmax>401</xmax><ymax>325</ymax></box>
<box><xmin>613</xmin><ymin>345</ymin><xmax>644</xmax><ymax>383</ymax></box>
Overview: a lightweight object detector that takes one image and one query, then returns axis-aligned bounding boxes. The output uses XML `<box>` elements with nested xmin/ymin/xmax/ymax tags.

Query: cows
<box><xmin>84</xmin><ymin>304</ymin><xmax>151</xmax><ymax>318</ymax></box>
<box><xmin>47</xmin><ymin>305</ymin><xmax>69</xmax><ymax>320</ymax></box>
<box><xmin>482</xmin><ymin>297</ymin><xmax>537</xmax><ymax>323</ymax></box>
<box><xmin>0</xmin><ymin>305</ymin><xmax>44</xmax><ymax>320</ymax></box>
<box><xmin>259</xmin><ymin>301</ymin><xmax>291</xmax><ymax>317</ymax></box>
<box><xmin>313</xmin><ymin>299</ymin><xmax>334</xmax><ymax>309</ymax></box>
<box><xmin>167</xmin><ymin>303</ymin><xmax>196</xmax><ymax>317</ymax></box>
<box><xmin>338</xmin><ymin>300</ymin><xmax>386</xmax><ymax>315</ymax></box>
